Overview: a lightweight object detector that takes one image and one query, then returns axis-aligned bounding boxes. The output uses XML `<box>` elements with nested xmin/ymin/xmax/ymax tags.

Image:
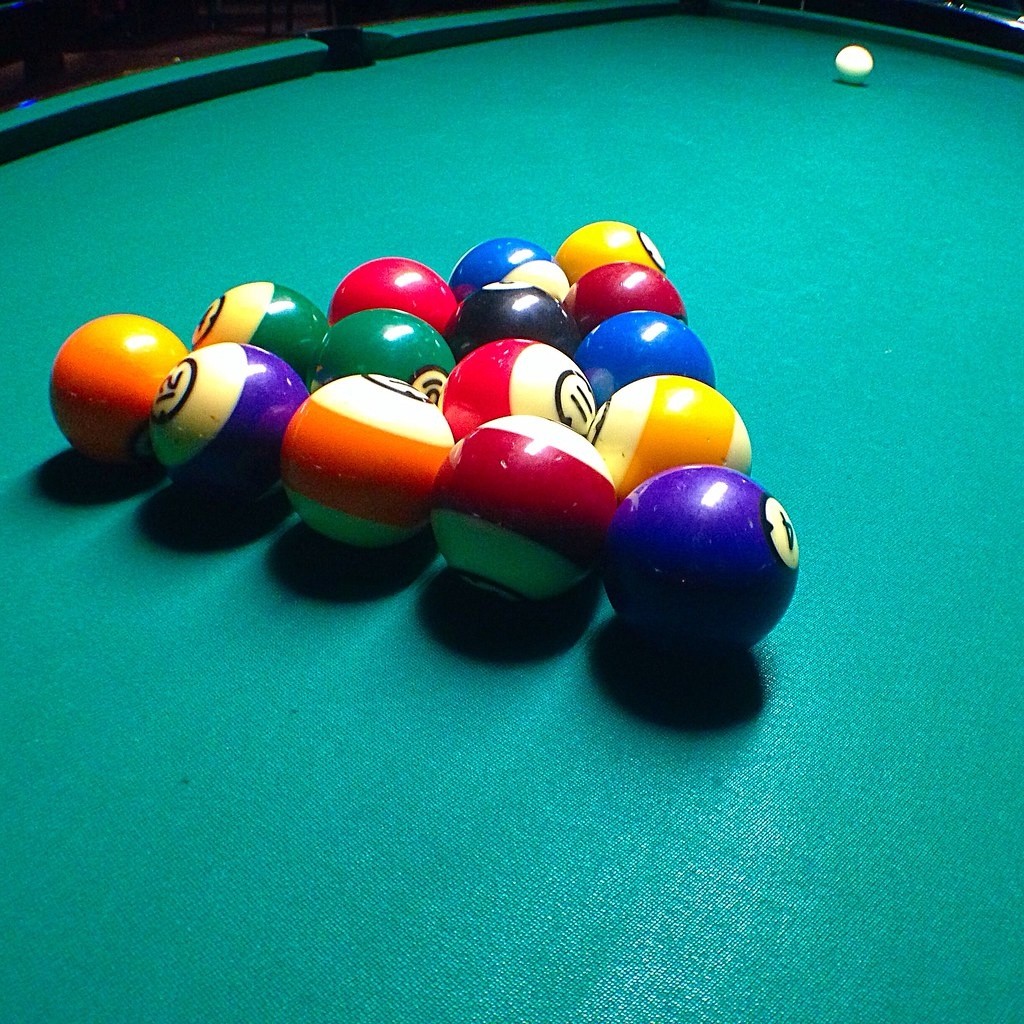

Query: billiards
<box><xmin>834</xmin><ymin>43</ymin><xmax>875</xmax><ymax>84</ymax></box>
<box><xmin>49</xmin><ymin>219</ymin><xmax>798</xmax><ymax>653</ymax></box>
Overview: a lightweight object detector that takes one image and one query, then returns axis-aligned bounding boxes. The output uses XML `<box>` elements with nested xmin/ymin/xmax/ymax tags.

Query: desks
<box><xmin>0</xmin><ymin>0</ymin><xmax>1024</xmax><ymax>1024</ymax></box>
